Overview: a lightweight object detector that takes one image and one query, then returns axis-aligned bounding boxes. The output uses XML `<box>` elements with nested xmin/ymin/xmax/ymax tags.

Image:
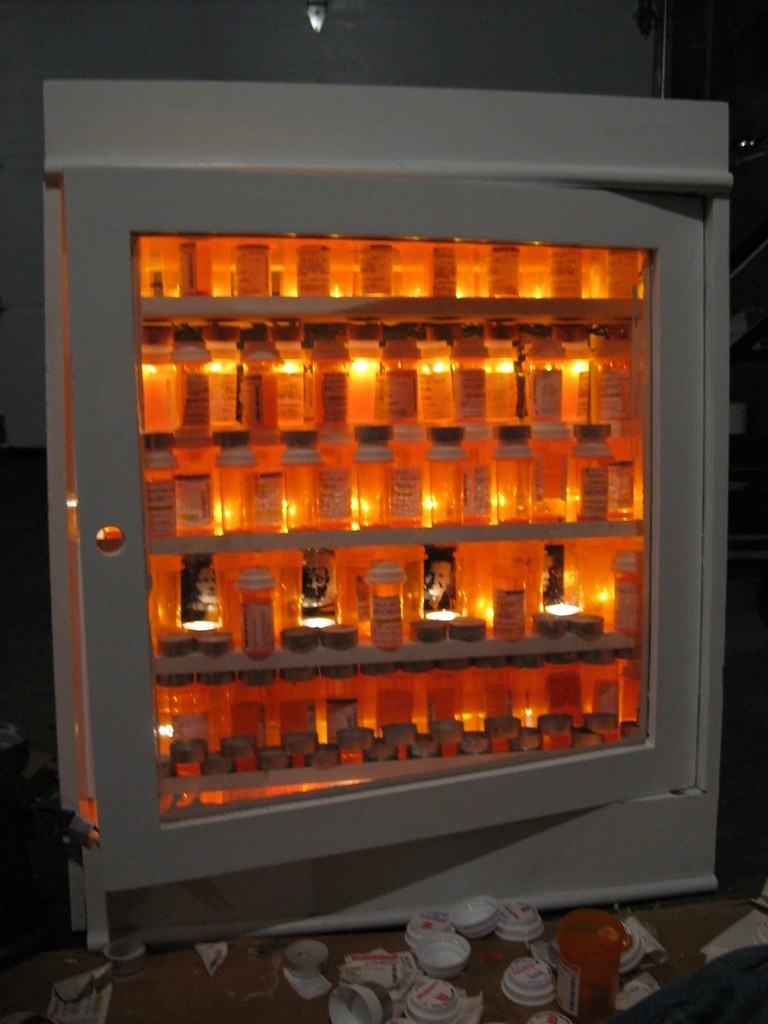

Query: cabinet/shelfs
<box><xmin>41</xmin><ymin>78</ymin><xmax>735</xmax><ymax>954</ymax></box>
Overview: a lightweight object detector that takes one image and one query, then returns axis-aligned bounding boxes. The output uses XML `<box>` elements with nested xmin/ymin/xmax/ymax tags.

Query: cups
<box><xmin>556</xmin><ymin>909</ymin><xmax>627</xmax><ymax>1019</ymax></box>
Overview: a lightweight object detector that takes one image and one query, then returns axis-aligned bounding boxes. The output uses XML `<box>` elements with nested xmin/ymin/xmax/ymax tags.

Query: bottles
<box><xmin>135</xmin><ymin>235</ymin><xmax>650</xmax><ymax>822</ymax></box>
